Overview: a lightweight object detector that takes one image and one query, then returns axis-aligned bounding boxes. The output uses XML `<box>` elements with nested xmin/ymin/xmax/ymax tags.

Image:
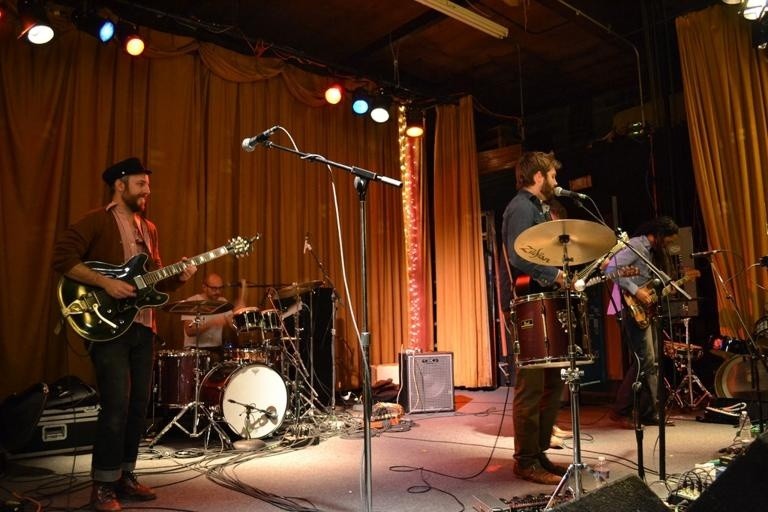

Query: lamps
<box><xmin>313</xmin><ymin>73</ymin><xmax>429</xmax><ymax>138</ymax></box>
<box><xmin>1</xmin><ymin>0</ymin><xmax>144</xmax><ymax>60</ymax></box>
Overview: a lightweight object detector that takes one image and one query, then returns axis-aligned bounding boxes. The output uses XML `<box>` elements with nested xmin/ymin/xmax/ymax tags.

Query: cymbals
<box><xmin>514</xmin><ymin>220</ymin><xmax>616</xmax><ymax>268</ymax></box>
<box><xmin>665</xmin><ymin>340</ymin><xmax>702</xmax><ymax>352</ymax></box>
<box><xmin>278</xmin><ymin>281</ymin><xmax>323</xmax><ymax>301</ymax></box>
<box><xmin>162</xmin><ymin>300</ymin><xmax>233</xmax><ymax>313</ymax></box>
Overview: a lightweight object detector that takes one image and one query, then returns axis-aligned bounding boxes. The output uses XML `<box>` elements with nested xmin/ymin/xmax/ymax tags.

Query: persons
<box><xmin>603</xmin><ymin>217</ymin><xmax>701</xmax><ymax>427</ymax></box>
<box><xmin>56</xmin><ymin>154</ymin><xmax>198</xmax><ymax>511</ymax></box>
<box><xmin>503</xmin><ymin>149</ymin><xmax>579</xmax><ymax>485</ymax></box>
<box><xmin>183</xmin><ymin>273</ymin><xmax>232</xmax><ymax>304</ymax></box>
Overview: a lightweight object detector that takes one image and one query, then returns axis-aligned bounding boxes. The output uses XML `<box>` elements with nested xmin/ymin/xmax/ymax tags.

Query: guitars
<box><xmin>57</xmin><ymin>231</ymin><xmax>258</xmax><ymax>340</ymax></box>
<box><xmin>624</xmin><ymin>271</ymin><xmax>700</xmax><ymax>329</ymax></box>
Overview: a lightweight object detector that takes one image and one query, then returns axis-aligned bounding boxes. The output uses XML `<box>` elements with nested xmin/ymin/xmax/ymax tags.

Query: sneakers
<box><xmin>608</xmin><ymin>409</ymin><xmax>644</xmax><ymax>430</ymax></box>
<box><xmin>91</xmin><ymin>483</ymin><xmax>122</xmax><ymax>512</ymax></box>
<box><xmin>550</xmin><ymin>424</ymin><xmax>572</xmax><ymax>448</ymax></box>
<box><xmin>644</xmin><ymin>416</ymin><xmax>674</xmax><ymax>425</ymax></box>
<box><xmin>512</xmin><ymin>460</ymin><xmax>565</xmax><ymax>484</ymax></box>
<box><xmin>117</xmin><ymin>471</ymin><xmax>156</xmax><ymax>500</ymax></box>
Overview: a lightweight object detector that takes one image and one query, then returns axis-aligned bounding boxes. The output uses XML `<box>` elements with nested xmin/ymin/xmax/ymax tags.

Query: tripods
<box><xmin>149</xmin><ymin>315</ymin><xmax>232</xmax><ymax>448</ymax></box>
<box><xmin>270</xmin><ymin>286</ymin><xmax>353</xmax><ymax>443</ymax></box>
<box><xmin>544</xmin><ymin>245</ymin><xmax>607</xmax><ymax>512</ymax></box>
<box><xmin>664</xmin><ymin>319</ymin><xmax>712</xmax><ymax>410</ymax></box>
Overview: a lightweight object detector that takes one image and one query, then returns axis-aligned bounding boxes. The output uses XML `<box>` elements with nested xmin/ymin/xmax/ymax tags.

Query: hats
<box><xmin>103</xmin><ymin>157</ymin><xmax>152</xmax><ymax>185</ymax></box>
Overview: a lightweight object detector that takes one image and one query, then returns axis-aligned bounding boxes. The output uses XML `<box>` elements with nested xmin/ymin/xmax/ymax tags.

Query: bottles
<box><xmin>594</xmin><ymin>457</ymin><xmax>611</xmax><ymax>490</ymax></box>
<box><xmin>739</xmin><ymin>409</ymin><xmax>751</xmax><ymax>441</ymax></box>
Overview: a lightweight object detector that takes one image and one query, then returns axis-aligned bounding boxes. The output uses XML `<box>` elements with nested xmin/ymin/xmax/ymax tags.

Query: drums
<box><xmin>229</xmin><ymin>305</ymin><xmax>260</xmax><ymax>331</ymax></box>
<box><xmin>259</xmin><ymin>310</ymin><xmax>281</xmax><ymax>331</ymax></box>
<box><xmin>708</xmin><ymin>315</ymin><xmax>767</xmax><ymax>399</ymax></box>
<box><xmin>155</xmin><ymin>348</ymin><xmax>210</xmax><ymax>405</ymax></box>
<box><xmin>199</xmin><ymin>347</ymin><xmax>288</xmax><ymax>438</ymax></box>
<box><xmin>509</xmin><ymin>292</ymin><xmax>594</xmax><ymax>369</ymax></box>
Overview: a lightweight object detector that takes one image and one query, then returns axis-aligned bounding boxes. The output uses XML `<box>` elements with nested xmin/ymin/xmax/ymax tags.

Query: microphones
<box><xmin>553</xmin><ymin>186</ymin><xmax>587</xmax><ymax>200</ymax></box>
<box><xmin>241</xmin><ymin>126</ymin><xmax>279</xmax><ymax>152</ymax></box>
<box><xmin>690</xmin><ymin>250</ymin><xmax>718</xmax><ymax>259</ymax></box>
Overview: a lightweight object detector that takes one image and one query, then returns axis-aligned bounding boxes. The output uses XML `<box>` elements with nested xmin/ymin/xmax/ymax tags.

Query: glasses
<box><xmin>205</xmin><ymin>283</ymin><xmax>224</xmax><ymax>291</ymax></box>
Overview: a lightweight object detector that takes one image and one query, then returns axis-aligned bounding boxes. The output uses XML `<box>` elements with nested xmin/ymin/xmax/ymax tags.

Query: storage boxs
<box><xmin>12</xmin><ymin>405</ymin><xmax>99</xmax><ymax>458</ymax></box>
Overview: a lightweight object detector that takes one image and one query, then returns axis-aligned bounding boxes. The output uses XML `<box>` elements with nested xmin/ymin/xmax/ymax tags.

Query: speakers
<box><xmin>398</xmin><ymin>351</ymin><xmax>455</xmax><ymax>414</ymax></box>
<box><xmin>686</xmin><ymin>429</ymin><xmax>768</xmax><ymax>512</ymax></box>
<box><xmin>546</xmin><ymin>474</ymin><xmax>672</xmax><ymax>512</ymax></box>
